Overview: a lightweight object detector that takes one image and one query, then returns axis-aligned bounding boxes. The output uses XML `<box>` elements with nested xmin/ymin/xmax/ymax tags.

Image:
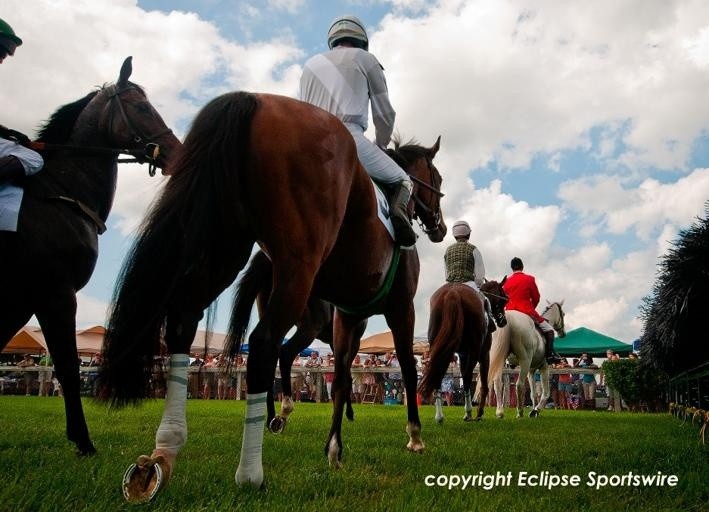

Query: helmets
<box><xmin>453</xmin><ymin>221</ymin><xmax>471</xmax><ymax>239</ymax></box>
<box><xmin>0</xmin><ymin>19</ymin><xmax>23</xmax><ymax>57</ymax></box>
<box><xmin>328</xmin><ymin>14</ymin><xmax>369</xmax><ymax>51</ymax></box>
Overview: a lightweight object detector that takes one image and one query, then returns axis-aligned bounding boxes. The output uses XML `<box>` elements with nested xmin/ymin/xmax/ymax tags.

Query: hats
<box><xmin>511</xmin><ymin>257</ymin><xmax>522</xmax><ymax>268</ymax></box>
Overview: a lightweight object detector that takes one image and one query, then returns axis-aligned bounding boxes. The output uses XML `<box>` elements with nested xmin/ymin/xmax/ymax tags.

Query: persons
<box><xmin>297</xmin><ymin>15</ymin><xmax>419</xmax><ymax>248</ymax></box>
<box><xmin>443</xmin><ymin>220</ymin><xmax>490</xmax><ymax>342</ymax></box>
<box><xmin>0</xmin><ymin>17</ymin><xmax>44</xmax><ymax>183</ymax></box>
<box><xmin>502</xmin><ymin>257</ymin><xmax>561</xmax><ymax>365</ymax></box>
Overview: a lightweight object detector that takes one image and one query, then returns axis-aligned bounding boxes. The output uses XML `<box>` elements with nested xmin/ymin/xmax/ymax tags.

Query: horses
<box><xmin>84</xmin><ymin>91</ymin><xmax>448</xmax><ymax>507</ymax></box>
<box><xmin>217</xmin><ymin>249</ymin><xmax>370</xmax><ymax>435</ymax></box>
<box><xmin>0</xmin><ymin>56</ymin><xmax>193</xmax><ymax>457</ymax></box>
<box><xmin>416</xmin><ymin>275</ymin><xmax>508</xmax><ymax>425</ymax></box>
<box><xmin>472</xmin><ymin>297</ymin><xmax>566</xmax><ymax>418</ymax></box>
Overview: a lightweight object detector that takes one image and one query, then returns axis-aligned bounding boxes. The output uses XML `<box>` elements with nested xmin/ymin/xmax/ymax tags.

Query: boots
<box><xmin>387</xmin><ymin>182</ymin><xmax>417</xmax><ymax>247</ymax></box>
<box><xmin>545</xmin><ymin>330</ymin><xmax>561</xmax><ymax>364</ymax></box>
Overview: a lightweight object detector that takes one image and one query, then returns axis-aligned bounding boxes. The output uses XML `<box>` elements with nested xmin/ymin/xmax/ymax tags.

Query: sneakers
<box><xmin>606</xmin><ymin>405</ymin><xmax>615</xmax><ymax>412</ymax></box>
